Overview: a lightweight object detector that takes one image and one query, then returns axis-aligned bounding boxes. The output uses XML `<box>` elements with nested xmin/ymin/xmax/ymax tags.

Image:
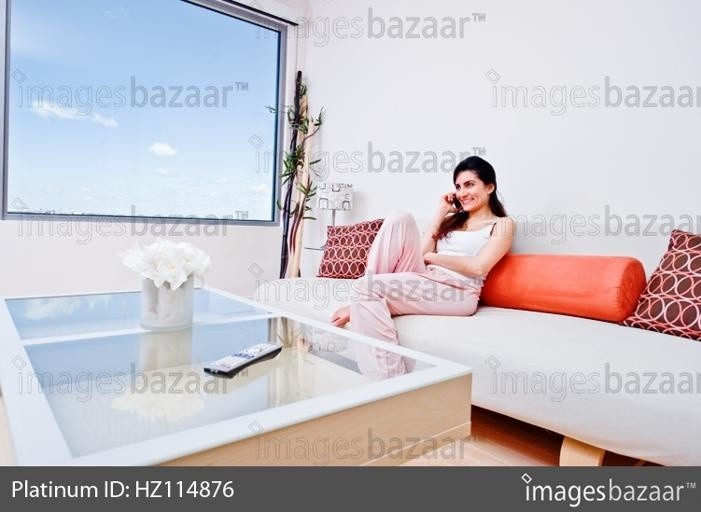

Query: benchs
<box><xmin>254</xmin><ymin>274</ymin><xmax>701</xmax><ymax>467</ymax></box>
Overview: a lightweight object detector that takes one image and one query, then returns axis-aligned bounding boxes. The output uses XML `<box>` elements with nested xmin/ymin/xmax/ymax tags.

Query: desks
<box><xmin>2</xmin><ymin>286</ymin><xmax>474</xmax><ymax>466</ymax></box>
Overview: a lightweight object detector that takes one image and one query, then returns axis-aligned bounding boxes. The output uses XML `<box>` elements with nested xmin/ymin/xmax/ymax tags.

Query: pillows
<box><xmin>314</xmin><ymin>217</ymin><xmax>384</xmax><ymax>279</ymax></box>
<box><xmin>481</xmin><ymin>228</ymin><xmax>701</xmax><ymax>343</ymax></box>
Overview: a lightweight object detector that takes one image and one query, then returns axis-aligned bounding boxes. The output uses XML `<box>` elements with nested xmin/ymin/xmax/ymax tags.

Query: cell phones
<box><xmin>452</xmin><ymin>193</ymin><xmax>461</xmax><ymax>210</ymax></box>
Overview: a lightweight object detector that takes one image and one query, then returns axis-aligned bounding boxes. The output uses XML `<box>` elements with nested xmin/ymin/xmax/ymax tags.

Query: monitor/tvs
<box><xmin>1</xmin><ymin>0</ymin><xmax>289</xmax><ymax>227</ymax></box>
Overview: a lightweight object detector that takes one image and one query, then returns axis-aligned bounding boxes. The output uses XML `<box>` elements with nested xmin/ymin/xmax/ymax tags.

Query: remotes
<box><xmin>203</xmin><ymin>341</ymin><xmax>284</xmax><ymax>377</ymax></box>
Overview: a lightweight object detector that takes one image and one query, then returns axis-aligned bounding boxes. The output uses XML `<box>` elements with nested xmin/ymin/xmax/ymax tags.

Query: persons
<box><xmin>327</xmin><ymin>152</ymin><xmax>517</xmax><ymax>382</ymax></box>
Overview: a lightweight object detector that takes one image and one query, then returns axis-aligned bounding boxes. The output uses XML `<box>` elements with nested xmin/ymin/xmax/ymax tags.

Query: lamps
<box><xmin>316</xmin><ymin>182</ymin><xmax>353</xmax><ymax>227</ymax></box>
